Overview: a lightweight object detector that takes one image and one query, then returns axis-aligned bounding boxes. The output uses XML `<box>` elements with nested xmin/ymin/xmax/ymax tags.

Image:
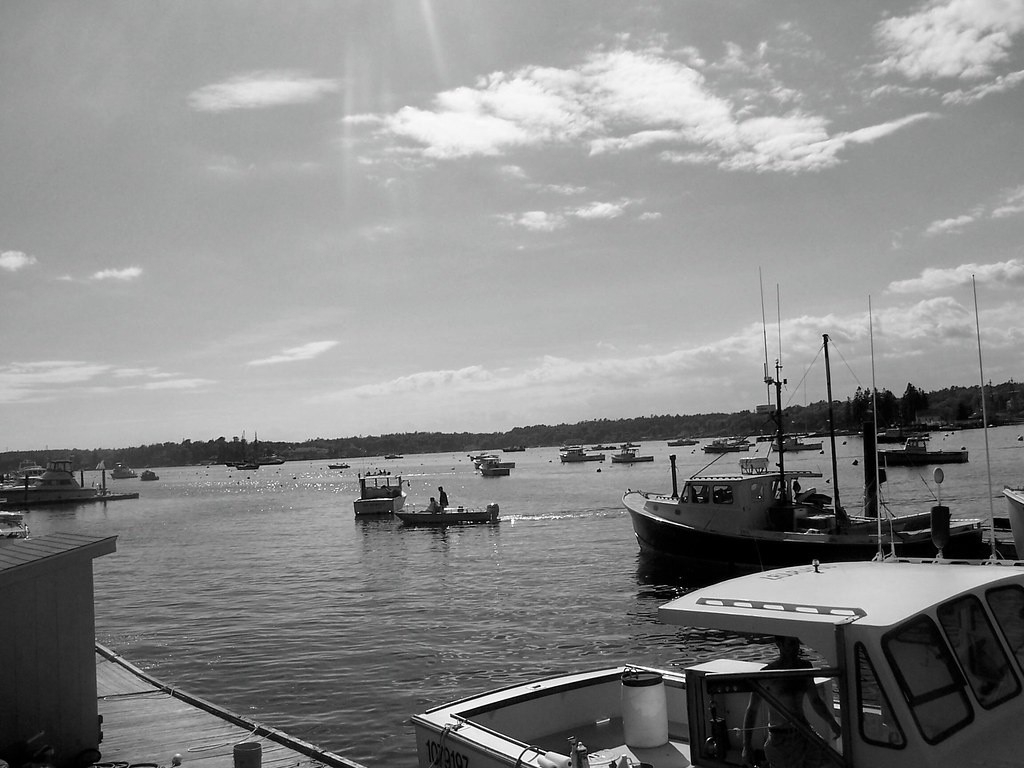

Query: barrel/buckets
<box><xmin>620</xmin><ymin>672</ymin><xmax>668</xmax><ymax>748</ymax></box>
<box><xmin>234</xmin><ymin>742</ymin><xmax>262</xmax><ymax>768</ymax></box>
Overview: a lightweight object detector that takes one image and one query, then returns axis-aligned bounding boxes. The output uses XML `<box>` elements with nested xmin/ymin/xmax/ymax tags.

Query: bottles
<box><xmin>576</xmin><ymin>742</ymin><xmax>590</xmax><ymax>768</ymax></box>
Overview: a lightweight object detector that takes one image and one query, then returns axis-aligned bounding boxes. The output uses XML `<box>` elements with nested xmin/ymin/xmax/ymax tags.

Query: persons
<box><xmin>742</xmin><ymin>635</ymin><xmax>843</xmax><ymax>768</ymax></box>
<box><xmin>419</xmin><ymin>496</ymin><xmax>435</xmax><ymax>513</ymax></box>
<box><xmin>438</xmin><ymin>486</ymin><xmax>449</xmax><ymax>511</ymax></box>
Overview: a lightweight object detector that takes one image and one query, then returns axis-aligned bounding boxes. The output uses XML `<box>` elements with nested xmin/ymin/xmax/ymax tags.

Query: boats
<box><xmin>385</xmin><ymin>453</ymin><xmax>404</xmax><ymax>459</ymax></box>
<box><xmin>619</xmin><ymin>264</ymin><xmax>988</xmax><ymax>569</ymax></box>
<box><xmin>470</xmin><ymin>454</ymin><xmax>515</xmax><ymax>476</ymax></box>
<box><xmin>353</xmin><ymin>469</ymin><xmax>411</xmax><ymax>515</ymax></box>
<box><xmin>771</xmin><ymin>438</ymin><xmax>822</xmax><ymax>451</ymax></box>
<box><xmin>328</xmin><ymin>463</ymin><xmax>350</xmax><ymax>469</ymax></box>
<box><xmin>756</xmin><ymin>434</ymin><xmax>791</xmax><ymax>442</ymax></box>
<box><xmin>502</xmin><ymin>445</ymin><xmax>526</xmax><ymax>452</ymax></box>
<box><xmin>404</xmin><ymin>272</ymin><xmax>1023</xmax><ymax>768</ymax></box>
<box><xmin>704</xmin><ymin>437</ymin><xmax>756</xmax><ymax>453</ymax></box>
<box><xmin>878</xmin><ymin>437</ymin><xmax>969</xmax><ymax>467</ymax></box>
<box><xmin>620</xmin><ymin>441</ymin><xmax>642</xmax><ymax>448</ymax></box>
<box><xmin>559</xmin><ymin>448</ymin><xmax>606</xmax><ymax>462</ymax></box>
<box><xmin>611</xmin><ymin>447</ymin><xmax>654</xmax><ymax>463</ymax></box>
<box><xmin>559</xmin><ymin>443</ymin><xmax>587</xmax><ymax>452</ymax></box>
<box><xmin>667</xmin><ymin>437</ymin><xmax>700</xmax><ymax>446</ymax></box>
<box><xmin>225</xmin><ymin>453</ymin><xmax>286</xmax><ymax>470</ymax></box>
<box><xmin>394</xmin><ymin>503</ymin><xmax>500</xmax><ymax>523</ymax></box>
<box><xmin>0</xmin><ymin>460</ymin><xmax>160</xmax><ymax>538</ymax></box>
<box><xmin>877</xmin><ymin>427</ymin><xmax>932</xmax><ymax>443</ymax></box>
<box><xmin>591</xmin><ymin>445</ymin><xmax>616</xmax><ymax>450</ymax></box>
